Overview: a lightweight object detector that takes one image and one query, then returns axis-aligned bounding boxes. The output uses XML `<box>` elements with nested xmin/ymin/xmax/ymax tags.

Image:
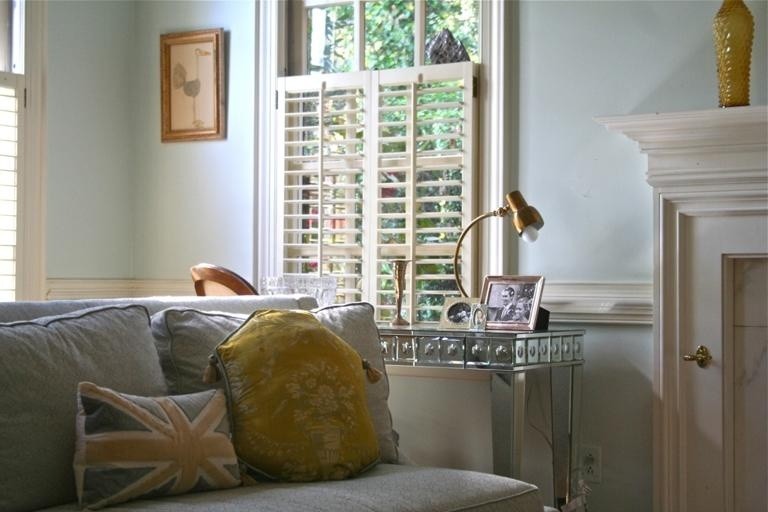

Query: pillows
<box><xmin>150</xmin><ymin>303</ymin><xmax>401</xmax><ymax>463</ymax></box>
<box><xmin>74</xmin><ymin>382</ymin><xmax>240</xmax><ymax>508</ymax></box>
<box><xmin>2</xmin><ymin>303</ymin><xmax>168</xmax><ymax>510</ymax></box>
<box><xmin>202</xmin><ymin>309</ymin><xmax>380</xmax><ymax>482</ymax></box>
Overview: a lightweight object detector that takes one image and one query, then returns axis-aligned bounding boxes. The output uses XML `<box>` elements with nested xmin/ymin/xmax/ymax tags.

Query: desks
<box><xmin>379</xmin><ymin>323</ymin><xmax>586</xmax><ymax>512</ymax></box>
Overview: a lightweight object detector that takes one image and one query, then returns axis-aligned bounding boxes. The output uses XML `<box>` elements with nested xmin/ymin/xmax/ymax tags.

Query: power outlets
<box><xmin>576</xmin><ymin>442</ymin><xmax>601</xmax><ymax>483</ymax></box>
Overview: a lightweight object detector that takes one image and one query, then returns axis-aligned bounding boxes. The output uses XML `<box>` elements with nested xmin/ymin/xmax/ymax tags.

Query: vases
<box><xmin>711</xmin><ymin>1</ymin><xmax>755</xmax><ymax>107</ymax></box>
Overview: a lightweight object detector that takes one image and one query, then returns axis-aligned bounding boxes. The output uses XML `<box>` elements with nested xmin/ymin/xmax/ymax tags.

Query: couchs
<box><xmin>0</xmin><ymin>294</ymin><xmax>545</xmax><ymax>512</ymax></box>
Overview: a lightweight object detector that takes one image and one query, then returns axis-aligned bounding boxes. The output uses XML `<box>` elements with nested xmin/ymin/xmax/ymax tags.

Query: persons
<box><xmin>513</xmin><ymin>296</ymin><xmax>532</xmax><ymax>320</ymax></box>
<box><xmin>494</xmin><ymin>286</ymin><xmax>516</xmax><ymax>320</ymax></box>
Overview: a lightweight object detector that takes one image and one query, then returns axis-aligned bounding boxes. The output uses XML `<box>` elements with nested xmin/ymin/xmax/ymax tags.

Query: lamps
<box><xmin>452</xmin><ymin>190</ymin><xmax>546</xmax><ymax>297</ymax></box>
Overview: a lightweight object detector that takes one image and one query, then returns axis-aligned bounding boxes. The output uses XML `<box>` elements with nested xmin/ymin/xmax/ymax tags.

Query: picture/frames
<box><xmin>159</xmin><ymin>28</ymin><xmax>226</xmax><ymax>143</ymax></box>
<box><xmin>478</xmin><ymin>274</ymin><xmax>546</xmax><ymax>332</ymax></box>
